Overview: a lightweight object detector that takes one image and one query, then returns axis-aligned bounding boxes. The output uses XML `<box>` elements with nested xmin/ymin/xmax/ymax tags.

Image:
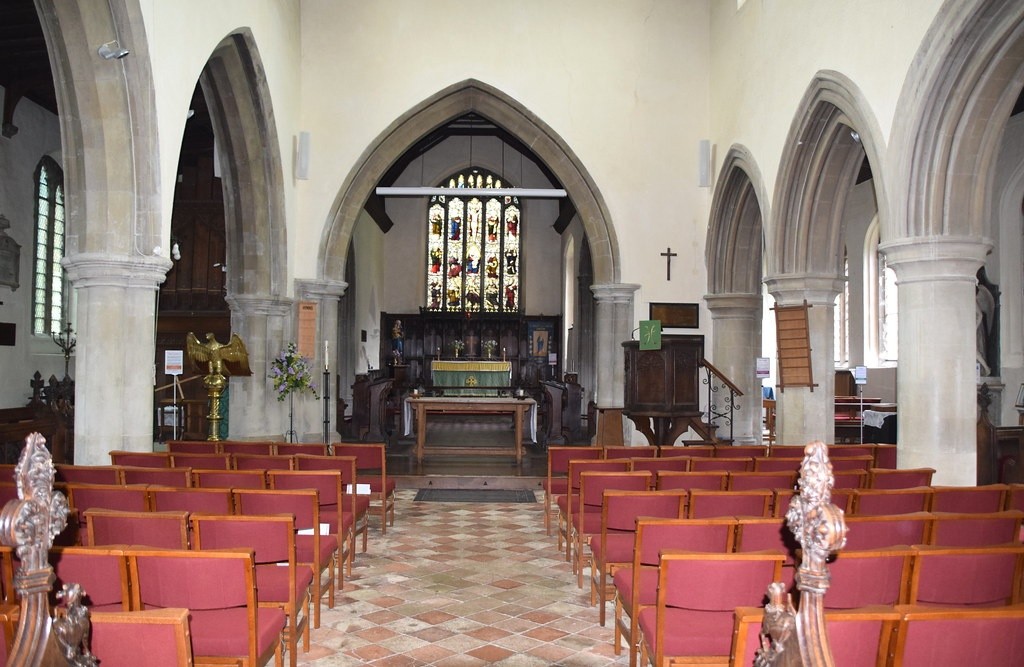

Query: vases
<box><xmin>454</xmin><ymin>348</ymin><xmax>458</xmax><ymax>358</ymax></box>
<box><xmin>487</xmin><ymin>349</ymin><xmax>491</xmax><ymax>358</ymax></box>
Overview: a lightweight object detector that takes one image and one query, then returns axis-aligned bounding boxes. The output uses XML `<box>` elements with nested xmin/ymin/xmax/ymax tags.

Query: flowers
<box><xmin>481</xmin><ymin>339</ymin><xmax>499</xmax><ymax>350</ymax></box>
<box><xmin>447</xmin><ymin>336</ymin><xmax>466</xmax><ymax>350</ymax></box>
<box><xmin>267</xmin><ymin>341</ymin><xmax>320</xmax><ymax>402</ymax></box>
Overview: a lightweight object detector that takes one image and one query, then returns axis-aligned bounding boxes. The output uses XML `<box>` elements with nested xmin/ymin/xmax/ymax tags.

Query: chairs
<box><xmin>542</xmin><ymin>443</ymin><xmax>1024</xmax><ymax>667</ymax></box>
<box><xmin>0</xmin><ymin>441</ymin><xmax>395</xmax><ymax>667</ymax></box>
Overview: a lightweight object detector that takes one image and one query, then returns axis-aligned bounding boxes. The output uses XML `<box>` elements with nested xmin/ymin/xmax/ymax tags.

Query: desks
<box><xmin>835</xmin><ymin>396</ymin><xmax>881</xmax><ymax>421</ymax></box>
<box><xmin>430</xmin><ymin>360</ymin><xmax>513</xmax><ymax>396</ymax></box>
<box><xmin>406</xmin><ymin>400</ymin><xmax>534</xmax><ymax>466</ymax></box>
